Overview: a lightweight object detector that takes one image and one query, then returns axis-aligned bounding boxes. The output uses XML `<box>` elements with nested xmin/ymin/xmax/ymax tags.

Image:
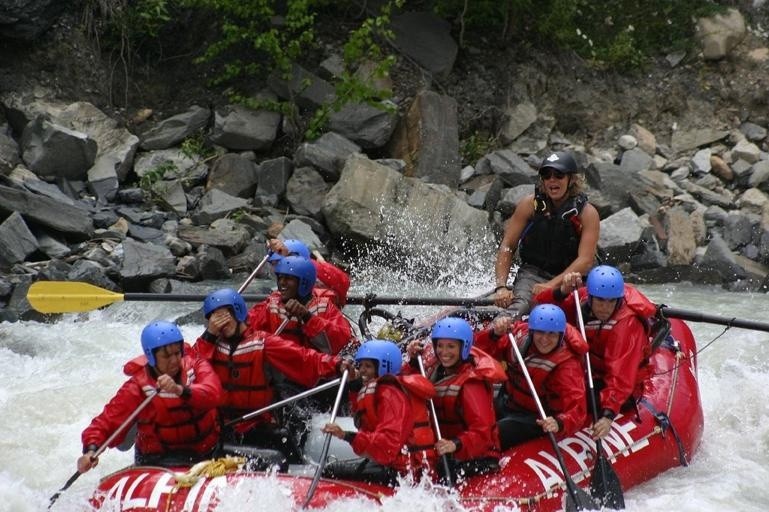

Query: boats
<box><xmin>82</xmin><ymin>276</ymin><xmax>706</xmax><ymax>512</ymax></box>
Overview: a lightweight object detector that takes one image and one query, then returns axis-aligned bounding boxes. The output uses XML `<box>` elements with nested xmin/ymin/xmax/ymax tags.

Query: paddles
<box><xmin>506</xmin><ymin>328</ymin><xmax>599</xmax><ymax>512</ymax></box>
<box><xmin>26</xmin><ymin>281</ymin><xmax>517</xmax><ymax>312</ymax></box>
<box><xmin>573</xmin><ymin>288</ymin><xmax>628</xmax><ymax>510</ymax></box>
<box><xmin>303</xmin><ymin>357</ymin><xmax>353</xmax><ymax>507</ymax></box>
<box><xmin>116</xmin><ymin>234</ymin><xmax>280</xmax><ymax>451</ymax></box>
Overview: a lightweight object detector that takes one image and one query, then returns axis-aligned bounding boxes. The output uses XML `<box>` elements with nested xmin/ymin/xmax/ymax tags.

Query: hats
<box><xmin>539</xmin><ymin>151</ymin><xmax>580</xmax><ymax>175</ymax></box>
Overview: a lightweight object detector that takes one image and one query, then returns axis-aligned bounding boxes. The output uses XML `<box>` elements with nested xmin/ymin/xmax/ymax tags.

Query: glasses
<box><xmin>539</xmin><ymin>169</ymin><xmax>566</xmax><ymax>179</ymax></box>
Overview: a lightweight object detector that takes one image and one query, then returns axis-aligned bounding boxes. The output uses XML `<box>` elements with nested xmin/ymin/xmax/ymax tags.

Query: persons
<box><xmin>320</xmin><ymin>337</ymin><xmax>436</xmax><ymax>488</ymax></box>
<box><xmin>490</xmin><ymin>152</ymin><xmax>599</xmax><ymax>323</ymax></box>
<box><xmin>403</xmin><ymin>316</ymin><xmax>501</xmax><ymax>484</ymax></box>
<box><xmin>264</xmin><ymin>238</ymin><xmax>350</xmax><ymax>311</ymax></box>
<box><xmin>474</xmin><ymin>303</ymin><xmax>587</xmax><ymax>459</ymax></box>
<box><xmin>533</xmin><ymin>266</ymin><xmax>657</xmax><ymax>440</ymax></box>
<box><xmin>78</xmin><ymin>319</ymin><xmax>220</xmax><ymax>474</ymax></box>
<box><xmin>194</xmin><ymin>288</ymin><xmax>343</xmax><ymax>464</ymax></box>
<box><xmin>243</xmin><ymin>258</ymin><xmax>352</xmax><ymax>354</ymax></box>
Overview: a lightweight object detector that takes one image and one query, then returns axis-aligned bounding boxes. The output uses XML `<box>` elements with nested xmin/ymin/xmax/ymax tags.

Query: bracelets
<box><xmin>494</xmin><ymin>286</ymin><xmax>508</xmax><ymax>291</ymax></box>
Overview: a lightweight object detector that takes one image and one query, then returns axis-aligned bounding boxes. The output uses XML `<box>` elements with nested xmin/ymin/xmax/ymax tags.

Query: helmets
<box><xmin>431</xmin><ymin>316</ymin><xmax>475</xmax><ymax>364</ymax></box>
<box><xmin>273</xmin><ymin>255</ymin><xmax>316</xmax><ymax>298</ymax></box>
<box><xmin>527</xmin><ymin>303</ymin><xmax>568</xmax><ymax>348</ymax></box>
<box><xmin>203</xmin><ymin>287</ymin><xmax>248</xmax><ymax>327</ymax></box>
<box><xmin>139</xmin><ymin>320</ymin><xmax>185</xmax><ymax>367</ymax></box>
<box><xmin>586</xmin><ymin>263</ymin><xmax>624</xmax><ymax>300</ymax></box>
<box><xmin>266</xmin><ymin>238</ymin><xmax>311</xmax><ymax>263</ymax></box>
<box><xmin>353</xmin><ymin>338</ymin><xmax>403</xmax><ymax>381</ymax></box>
